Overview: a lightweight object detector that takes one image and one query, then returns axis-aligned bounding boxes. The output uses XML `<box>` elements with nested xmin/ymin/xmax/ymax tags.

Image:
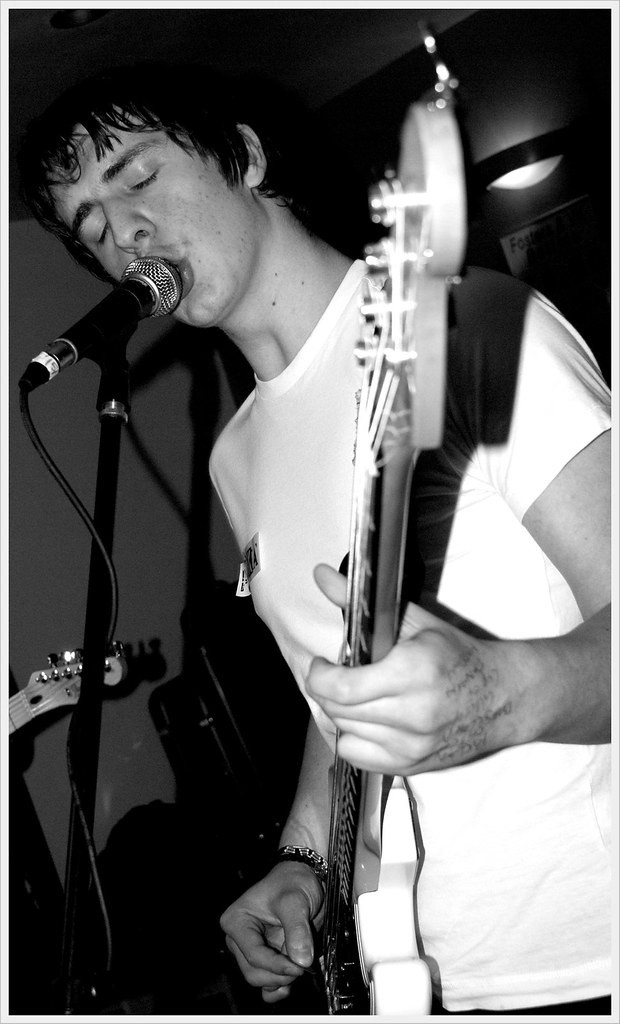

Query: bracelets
<box><xmin>273</xmin><ymin>844</ymin><xmax>328</xmax><ymax>883</ymax></box>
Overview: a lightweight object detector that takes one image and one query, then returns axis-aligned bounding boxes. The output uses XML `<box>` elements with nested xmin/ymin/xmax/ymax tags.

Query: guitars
<box><xmin>9</xmin><ymin>639</ymin><xmax>130</xmax><ymax>737</ymax></box>
<box><xmin>238</xmin><ymin>56</ymin><xmax>467</xmax><ymax>1015</ymax></box>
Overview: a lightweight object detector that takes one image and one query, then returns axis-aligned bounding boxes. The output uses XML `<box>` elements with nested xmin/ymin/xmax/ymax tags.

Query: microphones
<box><xmin>20</xmin><ymin>258</ymin><xmax>182</xmax><ymax>393</ymax></box>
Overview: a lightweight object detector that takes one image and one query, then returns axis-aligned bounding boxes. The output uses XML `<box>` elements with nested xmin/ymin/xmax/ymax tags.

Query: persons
<box><xmin>10</xmin><ymin>68</ymin><xmax>610</xmax><ymax>1015</ymax></box>
